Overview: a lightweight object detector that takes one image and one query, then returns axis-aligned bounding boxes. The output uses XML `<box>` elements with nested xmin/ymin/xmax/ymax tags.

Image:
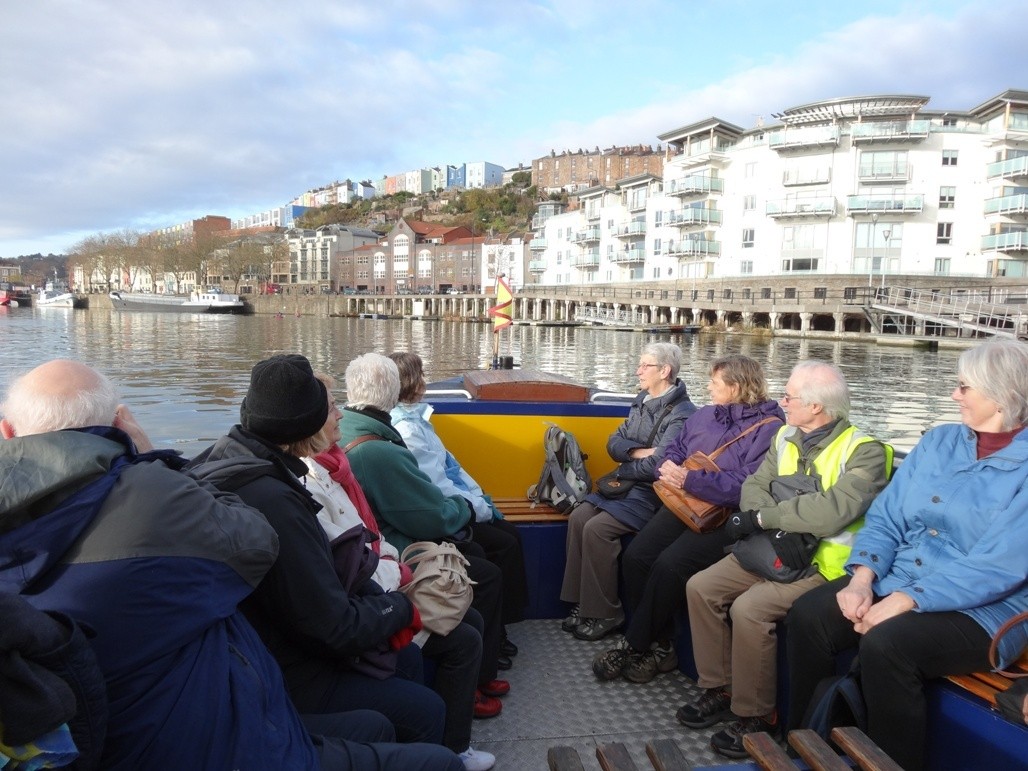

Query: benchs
<box><xmin>544</xmin><ymin>652</ymin><xmax>1028</xmax><ymax>771</ymax></box>
<box><xmin>426</xmin><ymin>409</ymin><xmax>658</xmax><ymax>605</ymax></box>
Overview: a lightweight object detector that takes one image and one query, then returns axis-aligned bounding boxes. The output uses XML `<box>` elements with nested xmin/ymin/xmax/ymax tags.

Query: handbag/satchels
<box><xmin>395</xmin><ymin>541</ymin><xmax>479</xmax><ymax>636</ymax></box>
<box><xmin>989</xmin><ymin>610</ymin><xmax>1028</xmax><ymax>728</ymax></box>
<box><xmin>653</xmin><ymin>450</ymin><xmax>732</xmax><ymax>534</ymax></box>
<box><xmin>596</xmin><ymin>471</ymin><xmax>638</xmax><ymax>500</ymax></box>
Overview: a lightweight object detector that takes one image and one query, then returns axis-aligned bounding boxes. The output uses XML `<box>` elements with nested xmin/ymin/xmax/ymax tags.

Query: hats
<box><xmin>239</xmin><ymin>354</ymin><xmax>329</xmax><ymax>441</ymax></box>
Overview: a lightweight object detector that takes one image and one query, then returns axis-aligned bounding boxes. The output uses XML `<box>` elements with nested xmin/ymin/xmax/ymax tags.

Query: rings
<box><xmin>842</xmin><ymin>607</ymin><xmax>846</xmax><ymax>611</ymax></box>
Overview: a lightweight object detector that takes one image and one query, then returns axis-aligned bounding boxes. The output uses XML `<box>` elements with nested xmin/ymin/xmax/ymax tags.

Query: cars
<box><xmin>445</xmin><ymin>287</ymin><xmax>461</xmax><ymax>294</ymax></box>
<box><xmin>207</xmin><ymin>288</ymin><xmax>224</xmax><ymax>294</ymax></box>
<box><xmin>322</xmin><ymin>288</ymin><xmax>339</xmax><ymax>295</ymax></box>
<box><xmin>415</xmin><ymin>285</ymin><xmax>439</xmax><ymax>294</ymax></box>
<box><xmin>398</xmin><ymin>287</ymin><xmax>413</xmax><ymax>295</ymax></box>
<box><xmin>340</xmin><ymin>287</ymin><xmax>358</xmax><ymax>295</ymax></box>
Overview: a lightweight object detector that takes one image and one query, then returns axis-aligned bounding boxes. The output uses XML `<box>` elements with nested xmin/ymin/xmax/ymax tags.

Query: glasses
<box><xmin>960</xmin><ymin>382</ymin><xmax>972</xmax><ymax>395</ymax></box>
<box><xmin>784</xmin><ymin>392</ymin><xmax>804</xmax><ymax>403</ymax></box>
<box><xmin>637</xmin><ymin>363</ymin><xmax>663</xmax><ymax>370</ymax></box>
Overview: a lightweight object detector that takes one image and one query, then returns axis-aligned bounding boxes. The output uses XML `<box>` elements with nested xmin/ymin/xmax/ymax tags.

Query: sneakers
<box><xmin>711</xmin><ymin>705</ymin><xmax>785</xmax><ymax>758</ymax></box>
<box><xmin>677</xmin><ymin>684</ymin><xmax>742</xmax><ymax>728</ymax></box>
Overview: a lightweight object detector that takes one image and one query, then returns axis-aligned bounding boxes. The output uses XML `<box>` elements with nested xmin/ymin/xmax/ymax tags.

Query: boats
<box><xmin>109</xmin><ymin>291</ymin><xmax>210</xmax><ymax>311</ymax></box>
<box><xmin>375</xmin><ymin>268</ymin><xmax>1027</xmax><ymax>771</ymax></box>
<box><xmin>190</xmin><ymin>291</ymin><xmax>244</xmax><ymax>313</ymax></box>
<box><xmin>35</xmin><ymin>289</ymin><xmax>78</xmax><ymax>308</ymax></box>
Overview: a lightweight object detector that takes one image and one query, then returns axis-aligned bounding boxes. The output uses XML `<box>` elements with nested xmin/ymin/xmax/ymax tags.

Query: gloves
<box><xmin>724</xmin><ymin>508</ymin><xmax>766</xmax><ymax>545</ymax></box>
<box><xmin>410</xmin><ymin>603</ymin><xmax>423</xmax><ymax>631</ymax></box>
<box><xmin>381</xmin><ymin>555</ymin><xmax>413</xmax><ymax>586</ymax></box>
<box><xmin>391</xmin><ymin>627</ymin><xmax>414</xmax><ymax>649</ymax></box>
<box><xmin>767</xmin><ymin>528</ymin><xmax>819</xmax><ymax>571</ymax></box>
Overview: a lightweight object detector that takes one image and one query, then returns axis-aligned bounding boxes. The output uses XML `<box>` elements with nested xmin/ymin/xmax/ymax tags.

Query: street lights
<box><xmin>469</xmin><ymin>216</ymin><xmax>487</xmax><ymax>292</ymax></box>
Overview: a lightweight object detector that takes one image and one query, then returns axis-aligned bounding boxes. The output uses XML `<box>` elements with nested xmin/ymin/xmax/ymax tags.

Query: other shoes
<box><xmin>562</xmin><ymin>604</ymin><xmax>591</xmax><ymax>632</ymax></box>
<box><xmin>500</xmin><ymin>640</ymin><xmax>518</xmax><ymax>656</ymax></box>
<box><xmin>618</xmin><ymin>635</ymin><xmax>679</xmax><ymax>671</ymax></box>
<box><xmin>458</xmin><ymin>747</ymin><xmax>496</xmax><ymax>771</ymax></box>
<box><xmin>573</xmin><ymin>610</ymin><xmax>625</xmax><ymax>640</ymax></box>
<box><xmin>593</xmin><ymin>647</ymin><xmax>656</xmax><ymax>682</ymax></box>
<box><xmin>496</xmin><ymin>654</ymin><xmax>512</xmax><ymax>670</ymax></box>
<box><xmin>472</xmin><ymin>689</ymin><xmax>502</xmax><ymax>719</ymax></box>
<box><xmin>486</xmin><ymin>680</ymin><xmax>509</xmax><ymax>697</ymax></box>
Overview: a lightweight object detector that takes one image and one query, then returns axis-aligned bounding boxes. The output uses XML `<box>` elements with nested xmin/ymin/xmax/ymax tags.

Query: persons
<box><xmin>384</xmin><ymin>348</ymin><xmax>530</xmax><ymax>628</ymax></box>
<box><xmin>311</xmin><ymin>367</ymin><xmax>510</xmax><ymax>722</ymax></box>
<box><xmin>781</xmin><ymin>335</ymin><xmax>1027</xmax><ymax>771</ymax></box>
<box><xmin>675</xmin><ymin>355</ymin><xmax>894</xmax><ymax>759</ymax></box>
<box><xmin>187</xmin><ymin>347</ymin><xmax>504</xmax><ymax>771</ymax></box>
<box><xmin>0</xmin><ymin>359</ymin><xmax>466</xmax><ymax>770</ymax></box>
<box><xmin>283</xmin><ymin>283</ymin><xmax>389</xmax><ymax>297</ymax></box>
<box><xmin>590</xmin><ymin>358</ymin><xmax>789</xmax><ymax>685</ymax></box>
<box><xmin>336</xmin><ymin>354</ymin><xmax>520</xmax><ymax>672</ymax></box>
<box><xmin>559</xmin><ymin>334</ymin><xmax>701</xmax><ymax>640</ymax></box>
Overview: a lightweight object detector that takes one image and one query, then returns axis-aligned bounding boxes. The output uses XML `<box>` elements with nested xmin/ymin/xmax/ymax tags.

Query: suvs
<box><xmin>259</xmin><ymin>283</ymin><xmax>282</xmax><ymax>294</ymax></box>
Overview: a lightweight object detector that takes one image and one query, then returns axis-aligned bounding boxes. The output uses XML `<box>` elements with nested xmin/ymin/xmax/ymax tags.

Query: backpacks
<box><xmin>725</xmin><ymin>456</ymin><xmax>830</xmax><ymax>583</ymax></box>
<box><xmin>527</xmin><ymin>420</ymin><xmax>593</xmax><ymax>515</ymax></box>
